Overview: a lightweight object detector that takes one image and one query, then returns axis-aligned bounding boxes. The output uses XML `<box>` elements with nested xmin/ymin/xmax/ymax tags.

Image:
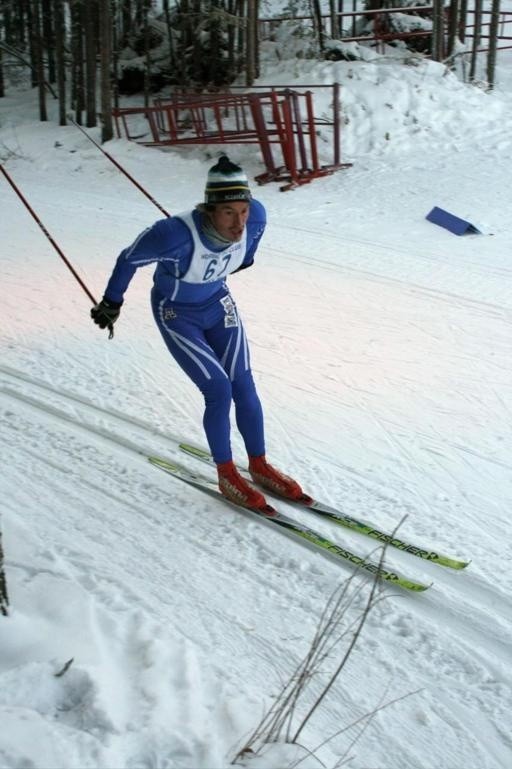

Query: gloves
<box><xmin>91</xmin><ymin>297</ymin><xmax>123</xmax><ymax>328</ymax></box>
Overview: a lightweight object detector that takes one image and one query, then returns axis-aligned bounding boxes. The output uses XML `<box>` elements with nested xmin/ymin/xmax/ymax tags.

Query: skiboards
<box><xmin>142</xmin><ymin>442</ymin><xmax>473</xmax><ymax>594</ymax></box>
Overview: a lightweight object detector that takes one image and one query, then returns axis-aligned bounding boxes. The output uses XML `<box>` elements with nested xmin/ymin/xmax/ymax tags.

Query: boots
<box><xmin>217</xmin><ymin>460</ymin><xmax>265</xmax><ymax>507</ymax></box>
<box><xmin>249</xmin><ymin>454</ymin><xmax>302</xmax><ymax>497</ymax></box>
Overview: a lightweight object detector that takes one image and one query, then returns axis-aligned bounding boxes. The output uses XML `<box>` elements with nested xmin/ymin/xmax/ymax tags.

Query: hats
<box><xmin>205</xmin><ymin>156</ymin><xmax>252</xmax><ymax>202</ymax></box>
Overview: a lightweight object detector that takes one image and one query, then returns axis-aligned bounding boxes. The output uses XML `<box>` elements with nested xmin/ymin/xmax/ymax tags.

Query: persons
<box><xmin>90</xmin><ymin>155</ymin><xmax>304</xmax><ymax>509</ymax></box>
